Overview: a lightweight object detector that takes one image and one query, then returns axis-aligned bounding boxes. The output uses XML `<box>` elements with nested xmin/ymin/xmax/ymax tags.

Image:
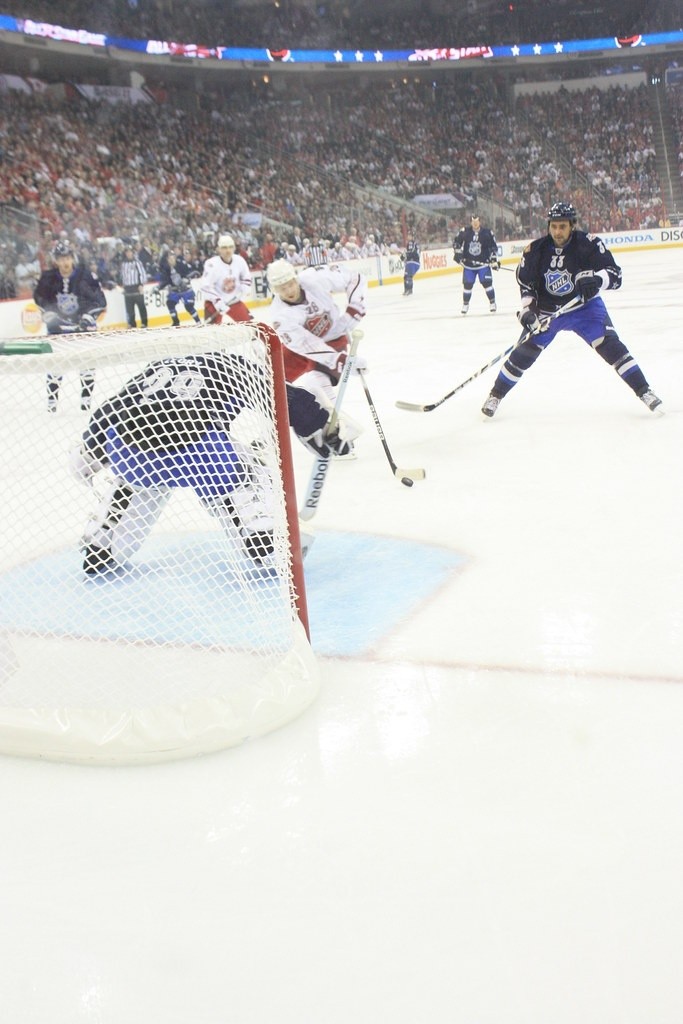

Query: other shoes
<box><xmin>81</xmin><ymin>396</ymin><xmax>91</xmax><ymax>412</ymax></box>
<box><xmin>47</xmin><ymin>400</ymin><xmax>57</xmax><ymax>412</ymax></box>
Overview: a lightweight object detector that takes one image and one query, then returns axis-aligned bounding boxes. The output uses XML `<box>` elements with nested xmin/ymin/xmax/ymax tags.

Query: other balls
<box><xmin>401</xmin><ymin>477</ymin><xmax>414</xmax><ymax>487</ymax></box>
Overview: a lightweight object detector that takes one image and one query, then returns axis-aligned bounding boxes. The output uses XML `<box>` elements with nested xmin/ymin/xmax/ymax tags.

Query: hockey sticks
<box><xmin>41</xmin><ymin>310</ymin><xmax>77</xmax><ymax>327</ymax></box>
<box><xmin>344</xmin><ymin>328</ymin><xmax>426</xmax><ymax>481</ymax></box>
<box><xmin>461</xmin><ymin>259</ymin><xmax>516</xmax><ymax>273</ymax></box>
<box><xmin>299</xmin><ymin>329</ymin><xmax>365</xmax><ymax>521</ymax></box>
<box><xmin>199</xmin><ymin>298</ymin><xmax>240</xmax><ymax>324</ymax></box>
<box><xmin>395</xmin><ymin>295</ymin><xmax>582</xmax><ymax>413</ymax></box>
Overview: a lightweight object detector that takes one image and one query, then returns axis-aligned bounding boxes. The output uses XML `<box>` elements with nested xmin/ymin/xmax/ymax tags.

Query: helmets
<box><xmin>266</xmin><ymin>260</ymin><xmax>296</xmax><ymax>286</ymax></box>
<box><xmin>217</xmin><ymin>235</ymin><xmax>236</xmax><ymax>249</ymax></box>
<box><xmin>470</xmin><ymin>214</ymin><xmax>480</xmax><ymax>224</ymax></box>
<box><xmin>546</xmin><ymin>203</ymin><xmax>577</xmax><ymax>227</ymax></box>
<box><xmin>54</xmin><ymin>241</ymin><xmax>72</xmax><ymax>257</ymax></box>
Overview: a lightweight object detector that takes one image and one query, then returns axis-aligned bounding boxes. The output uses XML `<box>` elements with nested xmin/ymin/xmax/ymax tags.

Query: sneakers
<box><xmin>82</xmin><ymin>543</ymin><xmax>115</xmax><ymax>574</ymax></box>
<box><xmin>461</xmin><ymin>304</ymin><xmax>469</xmax><ymax>314</ymax></box>
<box><xmin>640</xmin><ymin>389</ymin><xmax>665</xmax><ymax>415</ymax></box>
<box><xmin>490</xmin><ymin>302</ymin><xmax>496</xmax><ymax>312</ymax></box>
<box><xmin>481</xmin><ymin>392</ymin><xmax>500</xmax><ymax>421</ymax></box>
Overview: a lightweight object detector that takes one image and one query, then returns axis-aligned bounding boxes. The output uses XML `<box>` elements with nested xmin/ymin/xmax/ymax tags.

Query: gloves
<box><xmin>491</xmin><ymin>256</ymin><xmax>499</xmax><ymax>270</ymax></box>
<box><xmin>42</xmin><ymin>312</ymin><xmax>63</xmax><ymax>334</ymax></box>
<box><xmin>338</xmin><ymin>302</ymin><xmax>365</xmax><ymax>332</ymax></box>
<box><xmin>453</xmin><ymin>253</ymin><xmax>465</xmax><ymax>263</ymax></box>
<box><xmin>574</xmin><ymin>269</ymin><xmax>602</xmax><ymax>300</ymax></box>
<box><xmin>295</xmin><ymin>409</ymin><xmax>351</xmax><ymax>457</ymax></box>
<box><xmin>213</xmin><ymin>297</ymin><xmax>230</xmax><ymax>315</ymax></box>
<box><xmin>80</xmin><ymin>314</ymin><xmax>97</xmax><ymax>332</ymax></box>
<box><xmin>516</xmin><ymin>305</ymin><xmax>544</xmax><ymax>335</ymax></box>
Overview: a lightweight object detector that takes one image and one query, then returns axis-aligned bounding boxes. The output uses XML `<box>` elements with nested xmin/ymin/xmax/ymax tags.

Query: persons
<box><xmin>30</xmin><ymin>242</ymin><xmax>107</xmax><ymax>414</ymax></box>
<box><xmin>65</xmin><ymin>255</ymin><xmax>371</xmax><ymax>582</ymax></box>
<box><xmin>198</xmin><ymin>235</ymin><xmax>254</xmax><ymax>323</ymax></box>
<box><xmin>120</xmin><ymin>248</ymin><xmax>149</xmax><ymax>328</ymax></box>
<box><xmin>0</xmin><ymin>0</ymin><xmax>683</xmax><ymax>300</ymax></box>
<box><xmin>454</xmin><ymin>214</ymin><xmax>500</xmax><ymax>316</ymax></box>
<box><xmin>481</xmin><ymin>202</ymin><xmax>664</xmax><ymax>423</ymax></box>
<box><xmin>153</xmin><ymin>250</ymin><xmax>202</xmax><ymax>328</ymax></box>
<box><xmin>400</xmin><ymin>240</ymin><xmax>420</xmax><ymax>295</ymax></box>
